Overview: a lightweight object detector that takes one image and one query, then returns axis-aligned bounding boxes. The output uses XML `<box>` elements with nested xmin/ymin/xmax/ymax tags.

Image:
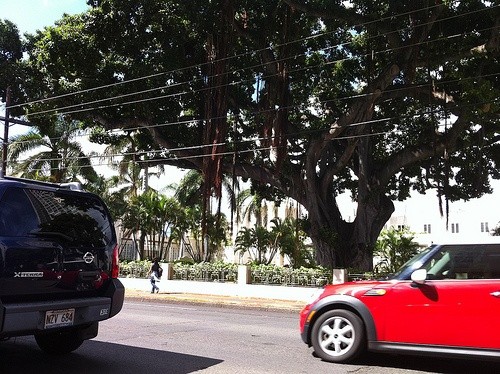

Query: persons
<box><xmin>146</xmin><ymin>257</ymin><xmax>162</xmax><ymax>293</ymax></box>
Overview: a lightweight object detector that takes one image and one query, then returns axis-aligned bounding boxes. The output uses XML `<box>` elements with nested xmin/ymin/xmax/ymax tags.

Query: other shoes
<box><xmin>156</xmin><ymin>288</ymin><xmax>159</xmax><ymax>293</ymax></box>
<box><xmin>151</xmin><ymin>291</ymin><xmax>154</xmax><ymax>293</ymax></box>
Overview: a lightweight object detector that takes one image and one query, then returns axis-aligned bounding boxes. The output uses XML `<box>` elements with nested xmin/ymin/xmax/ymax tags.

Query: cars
<box><xmin>300</xmin><ymin>242</ymin><xmax>500</xmax><ymax>363</ymax></box>
<box><xmin>44</xmin><ymin>269</ymin><xmax>67</xmax><ymax>281</ymax></box>
<box><xmin>97</xmin><ymin>269</ymin><xmax>108</xmax><ymax>281</ymax></box>
<box><xmin>77</xmin><ymin>263</ymin><xmax>100</xmax><ymax>281</ymax></box>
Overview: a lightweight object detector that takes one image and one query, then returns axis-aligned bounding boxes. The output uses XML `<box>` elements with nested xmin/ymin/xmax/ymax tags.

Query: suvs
<box><xmin>1</xmin><ymin>167</ymin><xmax>125</xmax><ymax>354</ymax></box>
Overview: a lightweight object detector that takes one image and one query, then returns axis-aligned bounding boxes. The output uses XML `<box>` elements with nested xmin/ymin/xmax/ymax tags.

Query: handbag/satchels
<box><xmin>158</xmin><ymin>267</ymin><xmax>163</xmax><ymax>277</ymax></box>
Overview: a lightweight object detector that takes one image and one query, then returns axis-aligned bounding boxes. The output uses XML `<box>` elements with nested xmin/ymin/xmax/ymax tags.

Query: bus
<box><xmin>47</xmin><ymin>260</ymin><xmax>94</xmax><ymax>274</ymax></box>
